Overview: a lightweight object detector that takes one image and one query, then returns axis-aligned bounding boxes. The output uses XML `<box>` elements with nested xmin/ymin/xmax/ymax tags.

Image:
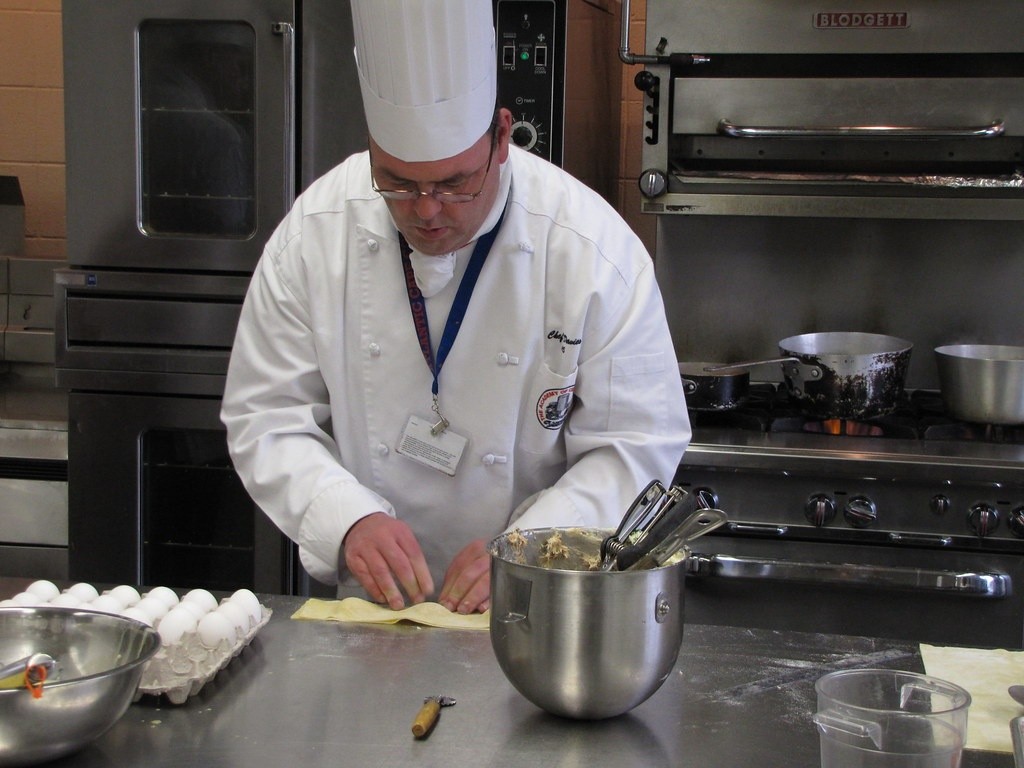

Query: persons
<box><xmin>220</xmin><ymin>0</ymin><xmax>693</xmax><ymax>616</ymax></box>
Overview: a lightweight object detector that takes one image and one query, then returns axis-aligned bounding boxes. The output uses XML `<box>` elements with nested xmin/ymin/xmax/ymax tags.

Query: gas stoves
<box><xmin>670</xmin><ymin>384</ymin><xmax>1024</xmax><ymax>556</ymax></box>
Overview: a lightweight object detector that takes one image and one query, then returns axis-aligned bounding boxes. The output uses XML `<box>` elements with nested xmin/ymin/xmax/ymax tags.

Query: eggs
<box><xmin>0</xmin><ymin>580</ymin><xmax>262</xmax><ymax>649</ymax></box>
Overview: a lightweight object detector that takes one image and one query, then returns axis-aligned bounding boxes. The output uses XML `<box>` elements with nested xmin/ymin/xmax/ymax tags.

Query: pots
<box><xmin>933</xmin><ymin>344</ymin><xmax>1024</xmax><ymax>425</ymax></box>
<box><xmin>676</xmin><ymin>362</ymin><xmax>751</xmax><ymax>410</ymax></box>
<box><xmin>701</xmin><ymin>331</ymin><xmax>914</xmax><ymax>420</ymax></box>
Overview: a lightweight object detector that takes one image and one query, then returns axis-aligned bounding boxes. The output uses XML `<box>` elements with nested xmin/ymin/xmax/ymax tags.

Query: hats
<box><xmin>349</xmin><ymin>0</ymin><xmax>498</xmax><ymax>163</ymax></box>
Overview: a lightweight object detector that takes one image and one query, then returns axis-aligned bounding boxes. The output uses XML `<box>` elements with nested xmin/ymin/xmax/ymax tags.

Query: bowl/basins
<box><xmin>484</xmin><ymin>526</ymin><xmax>689</xmax><ymax>721</ymax></box>
<box><xmin>0</xmin><ymin>606</ymin><xmax>162</xmax><ymax>768</ymax></box>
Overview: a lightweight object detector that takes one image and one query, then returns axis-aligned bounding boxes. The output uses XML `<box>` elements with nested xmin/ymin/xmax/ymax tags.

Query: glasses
<box><xmin>367</xmin><ymin>122</ymin><xmax>501</xmax><ymax>202</ymax></box>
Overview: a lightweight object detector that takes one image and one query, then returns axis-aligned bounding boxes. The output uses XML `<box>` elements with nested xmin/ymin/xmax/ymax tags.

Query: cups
<box><xmin>811</xmin><ymin>668</ymin><xmax>972</xmax><ymax>768</ymax></box>
